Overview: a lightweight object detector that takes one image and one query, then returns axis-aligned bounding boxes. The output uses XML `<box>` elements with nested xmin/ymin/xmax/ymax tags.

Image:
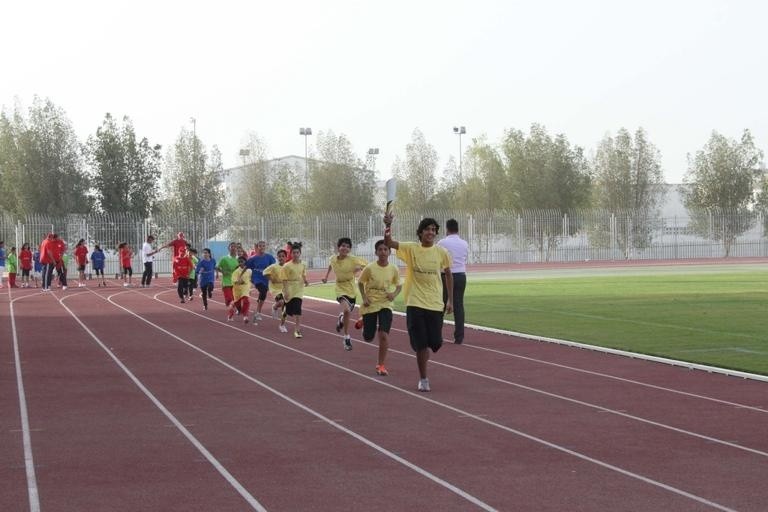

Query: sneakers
<box><xmin>354</xmin><ymin>314</ymin><xmax>365</xmax><ymax>330</ymax></box>
<box><xmin>9</xmin><ymin>279</ymin><xmax>155</xmax><ymax>294</ymax></box>
<box><xmin>278</xmin><ymin>321</ymin><xmax>289</xmax><ymax>333</ymax></box>
<box><xmin>293</xmin><ymin>327</ymin><xmax>303</xmax><ymax>338</ymax></box>
<box><xmin>271</xmin><ymin>303</ymin><xmax>279</xmax><ymax>320</ymax></box>
<box><xmin>176</xmin><ymin>293</ymin><xmax>213</xmax><ymax>311</ymax></box>
<box><xmin>455</xmin><ymin>339</ymin><xmax>464</xmax><ymax>344</ymax></box>
<box><xmin>336</xmin><ymin>311</ymin><xmax>346</xmax><ymax>333</ymax></box>
<box><xmin>417</xmin><ymin>376</ymin><xmax>432</xmax><ymax>392</ymax></box>
<box><xmin>341</xmin><ymin>334</ymin><xmax>353</xmax><ymax>353</ymax></box>
<box><xmin>225</xmin><ymin>306</ymin><xmax>263</xmax><ymax>326</ymax></box>
<box><xmin>375</xmin><ymin>360</ymin><xmax>389</xmax><ymax>376</ymax></box>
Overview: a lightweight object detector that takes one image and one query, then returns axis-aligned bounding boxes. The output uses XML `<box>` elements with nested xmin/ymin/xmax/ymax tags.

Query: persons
<box><xmin>1</xmin><ymin>231</ymin><xmax>68</xmax><ymax>291</ymax></box>
<box><xmin>160</xmin><ymin>232</ymin><xmax>311</xmax><ymax>340</ymax></box>
<box><xmin>140</xmin><ymin>235</ymin><xmax>158</xmax><ymax>288</ymax></box>
<box><xmin>73</xmin><ymin>238</ymin><xmax>106</xmax><ymax>288</ymax></box>
<box><xmin>322</xmin><ymin>212</ymin><xmax>470</xmax><ymax>393</ymax></box>
<box><xmin>113</xmin><ymin>242</ymin><xmax>137</xmax><ymax>287</ymax></box>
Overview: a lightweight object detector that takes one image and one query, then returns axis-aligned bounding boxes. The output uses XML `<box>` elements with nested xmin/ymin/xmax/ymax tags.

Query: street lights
<box><xmin>471</xmin><ymin>137</ymin><xmax>478</xmax><ymax>178</ymax></box>
<box><xmin>368</xmin><ymin>147</ymin><xmax>379</xmax><ymax>199</ymax></box>
<box><xmin>299</xmin><ymin>128</ymin><xmax>312</xmax><ymax>191</ymax></box>
<box><xmin>190</xmin><ymin>116</ymin><xmax>198</xmax><ymax>177</ymax></box>
<box><xmin>239</xmin><ymin>149</ymin><xmax>250</xmax><ymax>169</ymax></box>
<box><xmin>453</xmin><ymin>126</ymin><xmax>465</xmax><ymax>178</ymax></box>
